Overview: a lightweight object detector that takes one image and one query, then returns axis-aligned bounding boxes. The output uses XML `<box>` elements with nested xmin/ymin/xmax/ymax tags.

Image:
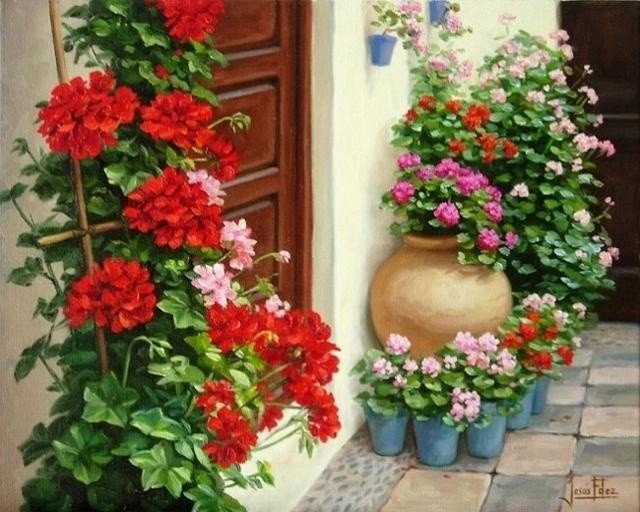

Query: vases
<box><xmin>370</xmin><ymin>34</ymin><xmax>398</xmax><ymax>67</ymax></box>
<box><xmin>370</xmin><ymin>409</ymin><xmax>408</xmax><ymax>455</ymax></box>
<box><xmin>464</xmin><ymin>403</ymin><xmax>507</xmax><ymax>458</ymax></box>
<box><xmin>431</xmin><ymin>1</ymin><xmax>450</xmax><ymax>27</ymax></box>
<box><xmin>370</xmin><ymin>233</ymin><xmax>513</xmax><ymax>359</ymax></box>
<box><xmin>414</xmin><ymin>418</ymin><xmax>459</xmax><ymax>466</ymax></box>
<box><xmin>535</xmin><ymin>376</ymin><xmax>551</xmax><ymax>414</ymax></box>
<box><xmin>510</xmin><ymin>381</ymin><xmax>533</xmax><ymax>429</ymax></box>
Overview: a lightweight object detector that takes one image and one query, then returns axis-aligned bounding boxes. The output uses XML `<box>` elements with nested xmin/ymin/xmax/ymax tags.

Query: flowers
<box><xmin>371</xmin><ymin>0</ymin><xmax>424</xmax><ymax>53</ymax></box>
<box><xmin>458</xmin><ymin>329</ymin><xmax>538</xmax><ymax>427</ymax></box>
<box><xmin>349</xmin><ymin>332</ymin><xmax>409</xmax><ymax>420</ymax></box>
<box><xmin>0</xmin><ymin>0</ymin><xmax>341</xmax><ymax>512</ymax></box>
<box><xmin>410</xmin><ymin>356</ymin><xmax>491</xmax><ymax>434</ymax></box>
<box><xmin>499</xmin><ymin>293</ymin><xmax>540</xmax><ymax>385</ymax></box>
<box><xmin>436</xmin><ymin>2</ymin><xmax>471</xmax><ymax>40</ymax></box>
<box><xmin>537</xmin><ymin>294</ymin><xmax>586</xmax><ymax>378</ymax></box>
<box><xmin>381</xmin><ymin>96</ymin><xmax>519</xmax><ymax>272</ymax></box>
